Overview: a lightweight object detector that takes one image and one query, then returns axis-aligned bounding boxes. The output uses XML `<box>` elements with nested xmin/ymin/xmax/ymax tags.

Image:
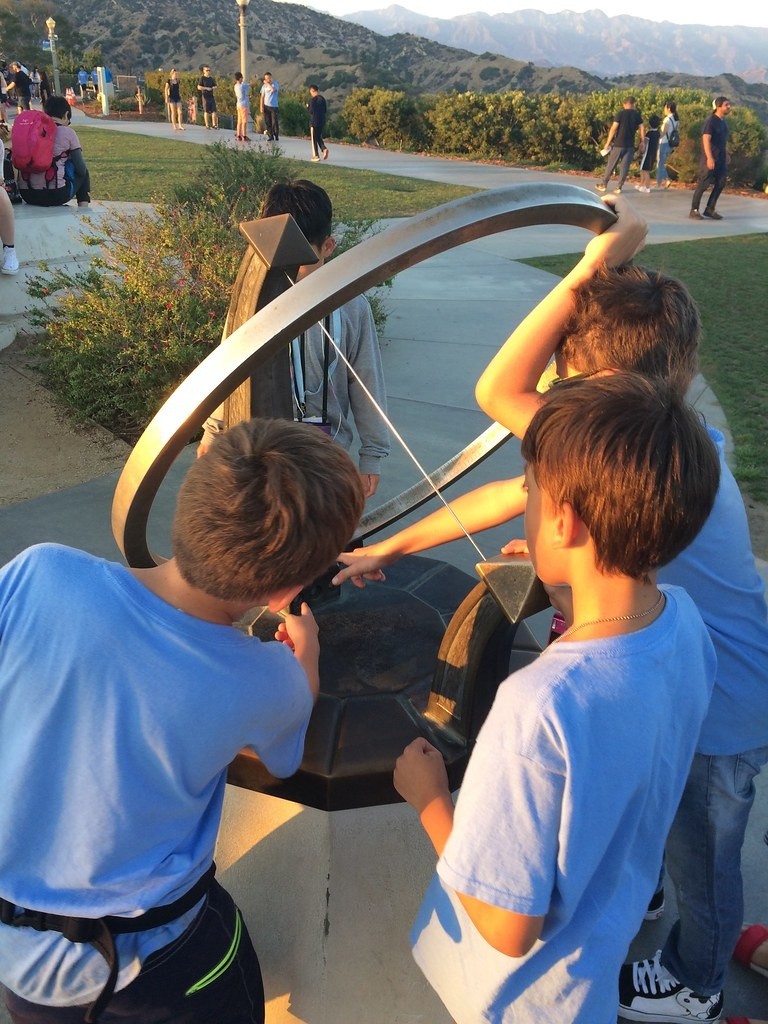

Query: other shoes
<box><xmin>596</xmin><ymin>184</ymin><xmax>606</xmax><ymax>191</ymax></box>
<box><xmin>322</xmin><ymin>149</ymin><xmax>329</xmax><ymax>159</ymax></box>
<box><xmin>614</xmin><ymin>186</ymin><xmax>622</xmax><ymax>193</ymax></box>
<box><xmin>635</xmin><ymin>185</ymin><xmax>650</xmax><ymax>193</ymax></box>
<box><xmin>703</xmin><ymin>208</ymin><xmax>723</xmax><ymax>219</ymax></box>
<box><xmin>241</xmin><ymin>136</ymin><xmax>251</xmax><ymax>142</ymax></box>
<box><xmin>664</xmin><ymin>180</ymin><xmax>672</xmax><ymax>190</ymax></box>
<box><xmin>689</xmin><ymin>210</ymin><xmax>705</xmax><ymax>221</ymax></box>
<box><xmin>311</xmin><ymin>156</ymin><xmax>319</xmax><ymax>161</ymax></box>
<box><xmin>236</xmin><ymin>136</ymin><xmax>241</xmax><ymax>141</ymax></box>
<box><xmin>651</xmin><ymin>184</ymin><xmax>662</xmax><ymax>190</ymax></box>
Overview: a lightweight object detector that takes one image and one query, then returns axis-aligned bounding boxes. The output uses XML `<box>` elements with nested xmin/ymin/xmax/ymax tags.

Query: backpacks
<box><xmin>7</xmin><ymin>109</ymin><xmax>70</xmax><ymax>182</ymax></box>
<box><xmin>666</xmin><ymin>116</ymin><xmax>680</xmax><ymax>148</ymax></box>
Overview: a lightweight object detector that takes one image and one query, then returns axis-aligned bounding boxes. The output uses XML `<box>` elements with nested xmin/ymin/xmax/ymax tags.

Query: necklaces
<box><xmin>539</xmin><ymin>589</ymin><xmax>663</xmax><ymax>656</ymax></box>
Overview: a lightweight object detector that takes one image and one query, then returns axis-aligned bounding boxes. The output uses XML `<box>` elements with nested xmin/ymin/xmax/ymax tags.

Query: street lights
<box><xmin>46</xmin><ymin>17</ymin><xmax>61</xmax><ymax>98</ymax></box>
<box><xmin>235</xmin><ymin>0</ymin><xmax>254</xmax><ymax>132</ymax></box>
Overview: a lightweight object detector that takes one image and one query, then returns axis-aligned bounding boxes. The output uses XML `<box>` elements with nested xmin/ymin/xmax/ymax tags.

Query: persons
<box><xmin>5</xmin><ymin>61</ymin><xmax>31</xmax><ymax>114</ymax></box>
<box><xmin>18</xmin><ymin>97</ymin><xmax>90</xmax><ymax>207</ymax></box>
<box><xmin>39</xmin><ymin>71</ymin><xmax>51</xmax><ymax>106</ymax></box>
<box><xmin>196</xmin><ymin>180</ymin><xmax>390</xmax><ymax>499</ymax></box>
<box><xmin>259</xmin><ymin>72</ymin><xmax>279</xmax><ymax>141</ymax></box>
<box><xmin>0</xmin><ymin>139</ymin><xmax>19</xmax><ymax>275</ymax></box>
<box><xmin>302</xmin><ymin>84</ymin><xmax>328</xmax><ymax>162</ymax></box>
<box><xmin>0</xmin><ymin>420</ymin><xmax>366</xmax><ymax>1024</ymax></box>
<box><xmin>234</xmin><ymin>72</ymin><xmax>252</xmax><ymax>141</ymax></box>
<box><xmin>78</xmin><ymin>65</ymin><xmax>89</xmax><ymax>93</ymax></box>
<box><xmin>197</xmin><ymin>65</ymin><xmax>218</xmax><ymax>129</ymax></box>
<box><xmin>31</xmin><ymin>66</ymin><xmax>41</xmax><ymax>99</ymax></box>
<box><xmin>650</xmin><ymin>101</ymin><xmax>678</xmax><ymax>191</ymax></box>
<box><xmin>0</xmin><ymin>71</ymin><xmax>8</xmax><ymax>123</ymax></box>
<box><xmin>91</xmin><ymin>65</ymin><xmax>98</xmax><ymax>90</ymax></box>
<box><xmin>335</xmin><ymin>194</ymin><xmax>768</xmax><ymax>1024</ymax></box>
<box><xmin>393</xmin><ymin>341</ymin><xmax>718</xmax><ymax>1024</ymax></box>
<box><xmin>634</xmin><ymin>116</ymin><xmax>660</xmax><ymax>193</ymax></box>
<box><xmin>689</xmin><ymin>97</ymin><xmax>730</xmax><ymax>220</ymax></box>
<box><xmin>164</xmin><ymin>68</ymin><xmax>186</xmax><ymax>130</ymax></box>
<box><xmin>594</xmin><ymin>96</ymin><xmax>646</xmax><ymax>193</ymax></box>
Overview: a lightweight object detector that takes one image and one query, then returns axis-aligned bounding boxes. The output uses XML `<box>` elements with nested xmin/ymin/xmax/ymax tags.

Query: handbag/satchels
<box><xmin>1</xmin><ymin>147</ymin><xmax>23</xmax><ymax>204</ymax></box>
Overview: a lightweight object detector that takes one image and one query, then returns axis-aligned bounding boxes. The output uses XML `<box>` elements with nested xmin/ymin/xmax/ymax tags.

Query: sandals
<box><xmin>733</xmin><ymin>922</ymin><xmax>768</xmax><ymax>976</ymax></box>
<box><xmin>720</xmin><ymin>1016</ymin><xmax>751</xmax><ymax>1024</ymax></box>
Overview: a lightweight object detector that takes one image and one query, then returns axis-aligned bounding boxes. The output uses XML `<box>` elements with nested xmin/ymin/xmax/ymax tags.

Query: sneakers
<box><xmin>2</xmin><ymin>246</ymin><xmax>19</xmax><ymax>275</ymax></box>
<box><xmin>621</xmin><ymin>949</ymin><xmax>724</xmax><ymax>1024</ymax></box>
<box><xmin>644</xmin><ymin>888</ymin><xmax>666</xmax><ymax>921</ymax></box>
